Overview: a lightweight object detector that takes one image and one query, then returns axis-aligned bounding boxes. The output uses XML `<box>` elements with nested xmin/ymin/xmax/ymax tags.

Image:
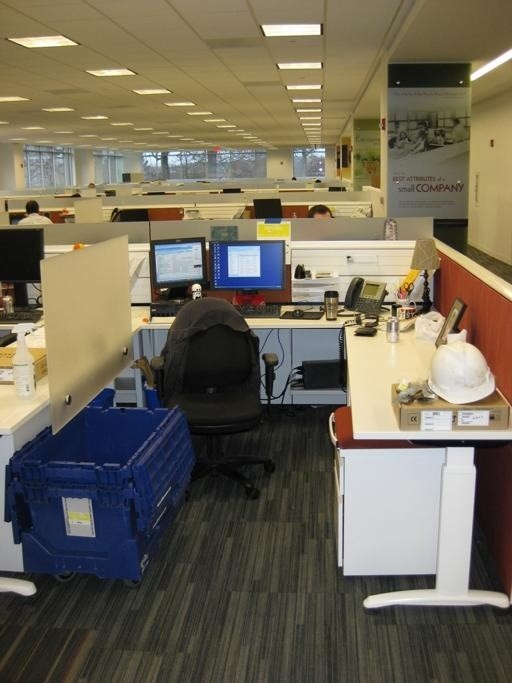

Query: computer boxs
<box><xmin>302</xmin><ymin>359</ymin><xmax>347</xmax><ymax>389</ymax></box>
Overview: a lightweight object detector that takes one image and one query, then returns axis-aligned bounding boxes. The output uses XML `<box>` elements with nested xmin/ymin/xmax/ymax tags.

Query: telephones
<box><xmin>344</xmin><ymin>277</ymin><xmax>387</xmax><ymax>314</ymax></box>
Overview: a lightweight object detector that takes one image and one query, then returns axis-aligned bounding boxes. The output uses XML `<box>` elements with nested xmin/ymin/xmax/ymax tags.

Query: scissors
<box><xmin>404</xmin><ymin>282</ymin><xmax>414</xmax><ymax>296</ymax></box>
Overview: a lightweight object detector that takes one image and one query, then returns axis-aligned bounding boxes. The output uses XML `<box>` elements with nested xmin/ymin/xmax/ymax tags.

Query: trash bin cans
<box><xmin>144</xmin><ymin>381</ymin><xmax>161</xmax><ymax>411</ymax></box>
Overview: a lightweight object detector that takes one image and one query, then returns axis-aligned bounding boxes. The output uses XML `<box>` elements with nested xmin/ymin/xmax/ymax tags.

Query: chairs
<box><xmin>149</xmin><ymin>307</ymin><xmax>278</xmax><ymax>500</ymax></box>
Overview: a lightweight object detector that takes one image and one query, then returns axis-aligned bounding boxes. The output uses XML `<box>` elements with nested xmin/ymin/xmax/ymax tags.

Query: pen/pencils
<box><xmin>396</xmin><ymin>287</ymin><xmax>401</xmax><ymax>299</ymax></box>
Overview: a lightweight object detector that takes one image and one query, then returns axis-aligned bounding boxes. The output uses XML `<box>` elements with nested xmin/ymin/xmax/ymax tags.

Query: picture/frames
<box><xmin>435</xmin><ymin>298</ymin><xmax>467</xmax><ymax>350</ymax></box>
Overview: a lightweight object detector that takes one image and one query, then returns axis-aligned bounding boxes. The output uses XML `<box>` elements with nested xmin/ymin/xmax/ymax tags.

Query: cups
<box><xmin>405</xmin><ymin>306</ymin><xmax>416</xmax><ymax>318</ymax></box>
<box><xmin>396</xmin><ymin>307</ymin><xmax>406</xmax><ymax>318</ymax></box>
<box><xmin>392</xmin><ymin>303</ymin><xmax>402</xmax><ymax>316</ymax></box>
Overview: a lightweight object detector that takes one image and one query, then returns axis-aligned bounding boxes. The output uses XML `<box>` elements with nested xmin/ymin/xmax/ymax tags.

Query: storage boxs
<box><xmin>4</xmin><ymin>405</ymin><xmax>197</xmax><ymax>588</ymax></box>
<box><xmin>392</xmin><ymin>383</ymin><xmax>511</xmax><ymax>432</ymax></box>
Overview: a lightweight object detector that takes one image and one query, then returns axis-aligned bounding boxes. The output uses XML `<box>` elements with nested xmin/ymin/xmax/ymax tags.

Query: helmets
<box><xmin>428</xmin><ymin>341</ymin><xmax>494</xmax><ymax>405</ymax></box>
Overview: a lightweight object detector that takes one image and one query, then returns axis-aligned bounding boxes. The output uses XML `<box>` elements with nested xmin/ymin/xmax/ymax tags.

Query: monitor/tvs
<box><xmin>253</xmin><ymin>199</ymin><xmax>282</xmax><ymax>218</ymax></box>
<box><xmin>0</xmin><ymin>228</ymin><xmax>45</xmax><ymax>311</ymax></box>
<box><xmin>210</xmin><ymin>240</ymin><xmax>285</xmax><ymax>294</ymax></box>
<box><xmin>120</xmin><ymin>209</ymin><xmax>148</xmax><ymax>222</ymax></box>
<box><xmin>149</xmin><ymin>236</ymin><xmax>207</xmax><ymax>300</ymax></box>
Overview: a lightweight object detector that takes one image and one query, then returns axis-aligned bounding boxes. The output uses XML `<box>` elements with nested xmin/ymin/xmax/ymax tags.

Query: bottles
<box><xmin>192</xmin><ymin>283</ymin><xmax>202</xmax><ymax>299</ymax></box>
<box><xmin>324</xmin><ymin>290</ymin><xmax>339</xmax><ymax>320</ymax></box>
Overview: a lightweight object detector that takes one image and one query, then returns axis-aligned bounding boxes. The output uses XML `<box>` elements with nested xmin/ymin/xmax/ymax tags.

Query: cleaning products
<box><xmin>11</xmin><ymin>323</ymin><xmax>37</xmax><ymax>399</ymax></box>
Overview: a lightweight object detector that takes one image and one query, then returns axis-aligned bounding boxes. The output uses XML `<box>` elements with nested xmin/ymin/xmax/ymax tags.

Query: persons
<box><xmin>16</xmin><ymin>199</ymin><xmax>54</xmax><ymax>225</ymax></box>
<box><xmin>88</xmin><ymin>183</ymin><xmax>95</xmax><ymax>188</ymax></box>
<box><xmin>307</xmin><ymin>203</ymin><xmax>334</xmax><ymax>219</ymax></box>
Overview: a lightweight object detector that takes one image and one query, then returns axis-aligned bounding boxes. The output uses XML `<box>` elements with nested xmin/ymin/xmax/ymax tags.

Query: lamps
<box><xmin>410</xmin><ymin>239</ymin><xmax>441</xmax><ymax>312</ymax></box>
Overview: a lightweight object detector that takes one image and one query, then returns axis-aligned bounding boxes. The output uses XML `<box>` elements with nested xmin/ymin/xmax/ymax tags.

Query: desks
<box><xmin>132</xmin><ymin>303</ymin><xmax>512</xmax><ymax>615</ymax></box>
<box><xmin>0</xmin><ymin>318</ymin><xmax>52</xmax><ymax>601</ymax></box>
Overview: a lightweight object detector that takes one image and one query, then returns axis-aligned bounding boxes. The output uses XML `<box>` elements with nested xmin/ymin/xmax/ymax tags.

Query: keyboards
<box><xmin>233</xmin><ymin>304</ymin><xmax>281</xmax><ymax>318</ymax></box>
<box><xmin>0</xmin><ymin>311</ymin><xmax>43</xmax><ymax>323</ymax></box>
<box><xmin>150</xmin><ymin>303</ymin><xmax>185</xmax><ymax>316</ymax></box>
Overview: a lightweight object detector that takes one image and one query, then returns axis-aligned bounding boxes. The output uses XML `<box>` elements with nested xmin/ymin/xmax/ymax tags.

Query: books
<box><xmin>0</xmin><ymin>346</ymin><xmax>49</xmax><ymax>385</ymax></box>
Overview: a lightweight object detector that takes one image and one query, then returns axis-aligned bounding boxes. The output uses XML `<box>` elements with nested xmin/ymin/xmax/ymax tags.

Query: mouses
<box><xmin>293</xmin><ymin>309</ymin><xmax>304</xmax><ymax>318</ymax></box>
